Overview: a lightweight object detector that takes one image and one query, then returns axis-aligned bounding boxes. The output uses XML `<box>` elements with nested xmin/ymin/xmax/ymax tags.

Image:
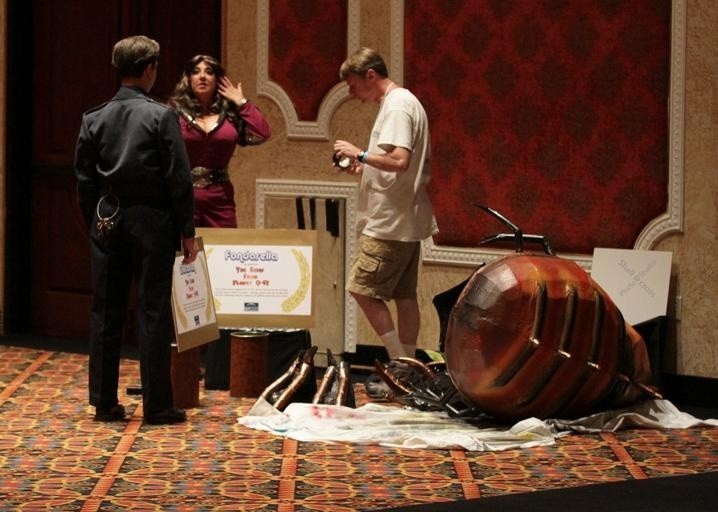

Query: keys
<box><xmin>96</xmin><ymin>217</ymin><xmax>113</xmax><ymax>232</ymax></box>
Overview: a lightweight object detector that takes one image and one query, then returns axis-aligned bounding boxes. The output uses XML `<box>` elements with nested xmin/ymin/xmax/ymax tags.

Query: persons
<box><xmin>74</xmin><ymin>35</ymin><xmax>198</xmax><ymax>424</ymax></box>
<box><xmin>168</xmin><ymin>54</ymin><xmax>272</xmax><ymax>389</ymax></box>
<box><xmin>333</xmin><ymin>47</ymin><xmax>440</xmax><ymax>401</ymax></box>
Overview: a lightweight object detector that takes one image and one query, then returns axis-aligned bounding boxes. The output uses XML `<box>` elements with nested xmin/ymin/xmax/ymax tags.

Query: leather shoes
<box><xmin>95</xmin><ymin>405</ymin><xmax>125</xmax><ymax>421</ymax></box>
<box><xmin>144</xmin><ymin>407</ymin><xmax>186</xmax><ymax>424</ymax></box>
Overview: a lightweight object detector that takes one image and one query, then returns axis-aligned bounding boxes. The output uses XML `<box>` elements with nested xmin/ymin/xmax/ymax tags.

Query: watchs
<box><xmin>238</xmin><ymin>98</ymin><xmax>248</xmax><ymax>107</ymax></box>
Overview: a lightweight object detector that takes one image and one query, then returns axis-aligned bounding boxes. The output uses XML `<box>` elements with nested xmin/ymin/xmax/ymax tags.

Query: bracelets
<box><xmin>358</xmin><ymin>150</ymin><xmax>368</xmax><ymax>162</ymax></box>
